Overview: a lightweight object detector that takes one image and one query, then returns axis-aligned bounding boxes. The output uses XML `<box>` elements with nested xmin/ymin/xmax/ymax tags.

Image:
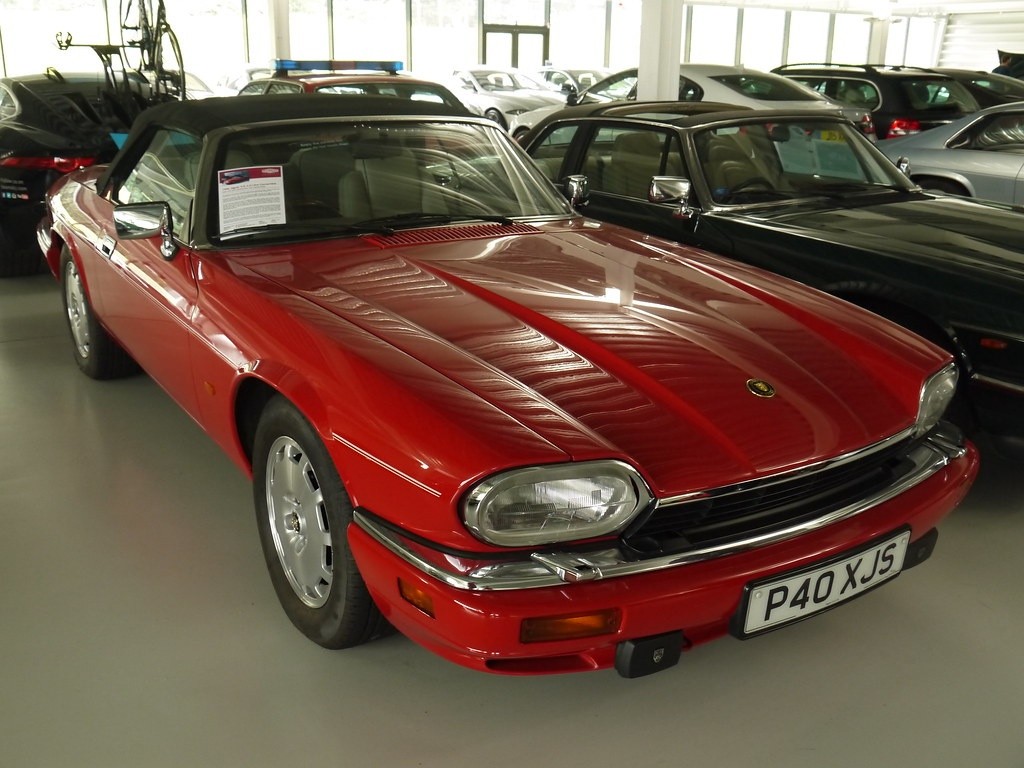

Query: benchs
<box><xmin>277</xmin><ymin>139</ymin><xmax>401</xmax><ymax>214</ymax></box>
<box><xmin>532</xmin><ymin>151</ymin><xmax>683</xmax><ymax>191</ymax></box>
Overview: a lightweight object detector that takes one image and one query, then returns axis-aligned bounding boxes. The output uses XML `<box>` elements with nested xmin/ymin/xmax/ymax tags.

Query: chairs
<box><xmin>602</xmin><ymin>132</ymin><xmax>676</xmax><ymax>201</ymax></box>
<box><xmin>338</xmin><ymin>148</ymin><xmax>449</xmax><ymax>222</ymax></box>
<box><xmin>913</xmin><ymin>86</ymin><xmax>929</xmax><ymax>104</ymax></box>
<box><xmin>163</xmin><ymin>150</ymin><xmax>298</xmax><ymax>236</ymax></box>
<box><xmin>844</xmin><ymin>90</ymin><xmax>865</xmax><ymax>105</ymax></box>
<box><xmin>702</xmin><ymin>133</ymin><xmax>795</xmax><ymax>201</ymax></box>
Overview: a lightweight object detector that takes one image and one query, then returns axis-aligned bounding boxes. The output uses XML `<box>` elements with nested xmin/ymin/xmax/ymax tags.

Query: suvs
<box><xmin>768</xmin><ymin>59</ymin><xmax>983</xmax><ymax>140</ymax></box>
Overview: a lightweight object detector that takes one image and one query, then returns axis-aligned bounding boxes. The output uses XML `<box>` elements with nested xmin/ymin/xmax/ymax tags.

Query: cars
<box><xmin>428</xmin><ymin>99</ymin><xmax>1024</xmax><ymax>435</ymax></box>
<box><xmin>410</xmin><ymin>63</ymin><xmax>568</xmax><ymax>132</ymax></box>
<box><xmin>228</xmin><ymin>58</ymin><xmax>494</xmax><ymax>194</ymax></box>
<box><xmin>0</xmin><ymin>68</ymin><xmax>199</xmax><ymax>278</ymax></box>
<box><xmin>35</xmin><ymin>93</ymin><xmax>982</xmax><ymax>679</ymax></box>
<box><xmin>507</xmin><ymin>64</ymin><xmax>879</xmax><ymax>157</ymax></box>
<box><xmin>903</xmin><ymin>67</ymin><xmax>1024</xmax><ymax>109</ymax></box>
<box><xmin>843</xmin><ymin>99</ymin><xmax>1024</xmax><ymax>209</ymax></box>
<box><xmin>524</xmin><ymin>63</ymin><xmax>636</xmax><ymax>106</ymax></box>
